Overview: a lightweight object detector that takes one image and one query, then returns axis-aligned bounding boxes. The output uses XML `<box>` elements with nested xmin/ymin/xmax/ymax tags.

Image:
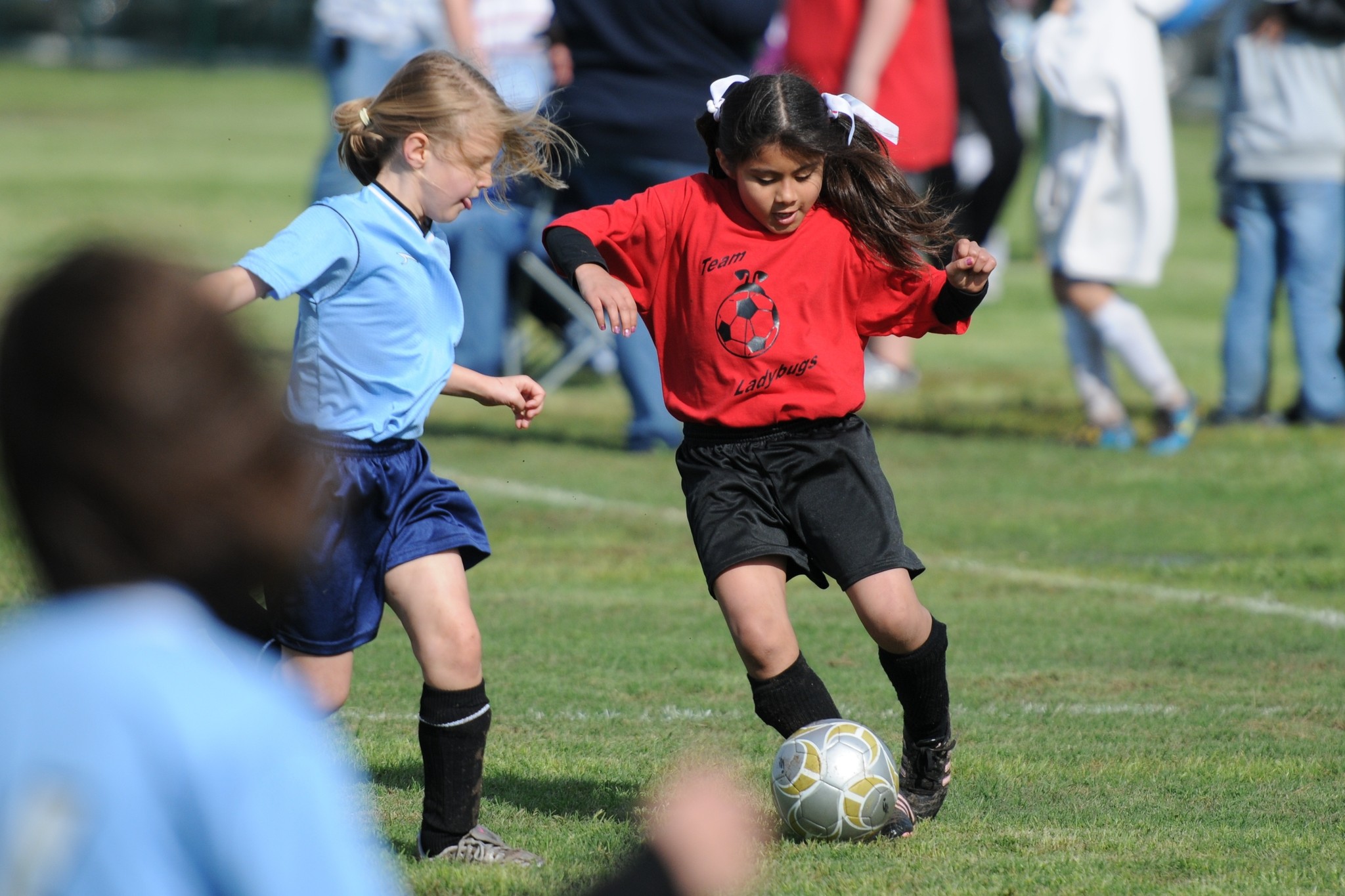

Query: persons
<box><xmin>1205</xmin><ymin>1</ymin><xmax>1344</xmax><ymax>425</ymax></box>
<box><xmin>542</xmin><ymin>73</ymin><xmax>997</xmax><ymax>824</ymax></box>
<box><xmin>186</xmin><ymin>50</ymin><xmax>589</xmax><ymax>866</ymax></box>
<box><xmin>0</xmin><ymin>238</ymin><xmax>407</xmax><ymax>894</ymax></box>
<box><xmin>1029</xmin><ymin>2</ymin><xmax>1202</xmax><ymax>456</ymax></box>
<box><xmin>304</xmin><ymin>1</ymin><xmax>1025</xmax><ymax>446</ymax></box>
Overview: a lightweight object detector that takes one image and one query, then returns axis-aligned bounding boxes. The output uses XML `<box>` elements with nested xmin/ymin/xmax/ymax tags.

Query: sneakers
<box><xmin>880</xmin><ymin>719</ymin><xmax>959</xmax><ymax>840</ymax></box>
<box><xmin>1274</xmin><ymin>398</ymin><xmax>1343</xmax><ymax>426</ymax></box>
<box><xmin>1145</xmin><ymin>393</ymin><xmax>1202</xmax><ymax>458</ymax></box>
<box><xmin>413</xmin><ymin>824</ymin><xmax>543</xmax><ymax>872</ymax></box>
<box><xmin>1202</xmin><ymin>401</ymin><xmax>1267</xmax><ymax>426</ymax></box>
<box><xmin>1069</xmin><ymin>420</ymin><xmax>1138</xmax><ymax>452</ymax></box>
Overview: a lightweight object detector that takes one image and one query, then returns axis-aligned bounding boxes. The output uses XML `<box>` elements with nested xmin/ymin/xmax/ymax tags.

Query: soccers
<box><xmin>772</xmin><ymin>719</ymin><xmax>898</xmax><ymax>841</ymax></box>
